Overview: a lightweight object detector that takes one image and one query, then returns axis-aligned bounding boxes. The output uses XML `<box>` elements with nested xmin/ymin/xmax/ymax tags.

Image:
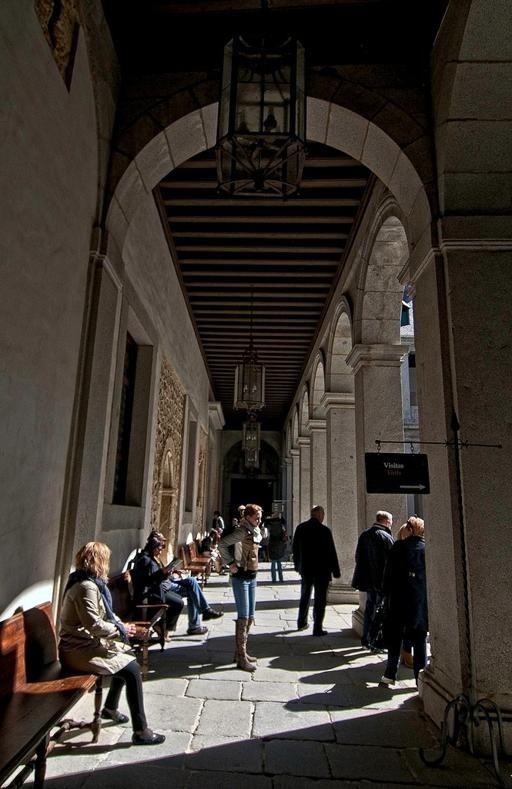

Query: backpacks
<box><xmin>219</xmin><ymin>525</ymin><xmax>253</xmax><ymax>572</ymax></box>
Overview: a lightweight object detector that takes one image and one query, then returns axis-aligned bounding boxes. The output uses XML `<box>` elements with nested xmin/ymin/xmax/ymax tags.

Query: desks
<box><xmin>107</xmin><ymin>607</ymin><xmax>164</xmax><ymax>676</ymax></box>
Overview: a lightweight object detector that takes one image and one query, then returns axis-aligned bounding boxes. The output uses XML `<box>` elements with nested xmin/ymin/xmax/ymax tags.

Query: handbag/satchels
<box><xmin>370</xmin><ymin>607</ymin><xmax>387</xmax><ymax>649</ymax></box>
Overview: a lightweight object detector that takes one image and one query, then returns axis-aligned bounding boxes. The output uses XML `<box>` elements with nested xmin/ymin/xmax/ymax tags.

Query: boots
<box><xmin>233</xmin><ymin>618</ymin><xmax>256</xmax><ymax>672</ymax></box>
<box><xmin>233</xmin><ymin>619</ymin><xmax>257</xmax><ymax>662</ymax></box>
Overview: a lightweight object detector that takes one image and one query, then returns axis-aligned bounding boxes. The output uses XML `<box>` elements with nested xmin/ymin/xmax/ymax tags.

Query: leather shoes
<box><xmin>362</xmin><ymin>643</ymin><xmax>371</xmax><ymax>650</ymax></box>
<box><xmin>313</xmin><ymin>630</ymin><xmax>327</xmax><ymax>637</ymax></box>
<box><xmin>132</xmin><ymin>728</ymin><xmax>165</xmax><ymax>744</ymax></box>
<box><xmin>298</xmin><ymin>623</ymin><xmax>308</xmax><ymax>631</ymax></box>
<box><xmin>101</xmin><ymin>709</ymin><xmax>129</xmax><ymax>722</ymax></box>
<box><xmin>371</xmin><ymin>647</ymin><xmax>388</xmax><ymax>654</ymax></box>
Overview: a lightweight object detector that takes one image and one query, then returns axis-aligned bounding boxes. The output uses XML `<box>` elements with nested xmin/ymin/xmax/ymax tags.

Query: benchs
<box><xmin>119</xmin><ymin>563</ymin><xmax>192</xmax><ymax>640</ymax></box>
<box><xmin>182</xmin><ymin>538</ymin><xmax>213</xmax><ymax>589</ymax></box>
<box><xmin>0</xmin><ymin>601</ymin><xmax>101</xmax><ymax>789</ymax></box>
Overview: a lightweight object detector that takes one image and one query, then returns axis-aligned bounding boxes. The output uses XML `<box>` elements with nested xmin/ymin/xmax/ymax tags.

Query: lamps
<box><xmin>233</xmin><ymin>288</ymin><xmax>265</xmax><ymax>410</ymax></box>
<box><xmin>241</xmin><ymin>412</ymin><xmax>261</xmax><ymax>470</ymax></box>
<box><xmin>213</xmin><ymin>0</ymin><xmax>309</xmax><ymax>200</ymax></box>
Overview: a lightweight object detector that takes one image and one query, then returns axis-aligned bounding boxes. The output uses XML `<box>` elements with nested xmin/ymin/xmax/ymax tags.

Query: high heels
<box><xmin>400</xmin><ymin>648</ymin><xmax>414</xmax><ymax>668</ymax></box>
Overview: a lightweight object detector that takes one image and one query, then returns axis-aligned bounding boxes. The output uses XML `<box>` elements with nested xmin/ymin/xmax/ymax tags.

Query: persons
<box><xmin>381</xmin><ymin>515</ymin><xmax>424</xmax><ymax>688</ymax></box>
<box><xmin>133</xmin><ymin>538</ymin><xmax>185</xmax><ymax>642</ymax></box>
<box><xmin>265</xmin><ymin>511</ymin><xmax>288</xmax><ymax>583</ymax></box>
<box><xmin>197</xmin><ymin>529</ymin><xmax>227</xmax><ymax>575</ymax></box>
<box><xmin>217</xmin><ymin>502</ymin><xmax>264</xmax><ymax>671</ymax></box>
<box><xmin>260</xmin><ymin>522</ymin><xmax>269</xmax><ymax>561</ymax></box>
<box><xmin>228</xmin><ymin>517</ymin><xmax>239</xmax><ymax>527</ymax></box>
<box><xmin>291</xmin><ymin>504</ymin><xmax>341</xmax><ymax>635</ymax></box>
<box><xmin>209</xmin><ymin>544</ymin><xmax>221</xmax><ymax>558</ymax></box>
<box><xmin>395</xmin><ymin>522</ymin><xmax>416</xmax><ymax>668</ymax></box>
<box><xmin>214</xmin><ymin>526</ymin><xmax>224</xmax><ymax>542</ymax></box>
<box><xmin>211</xmin><ymin>510</ymin><xmax>225</xmax><ymax>531</ymax></box>
<box><xmin>352</xmin><ymin>511</ymin><xmax>392</xmax><ymax>650</ymax></box>
<box><xmin>237</xmin><ymin>504</ymin><xmax>245</xmax><ymax>517</ymax></box>
<box><xmin>58</xmin><ymin>541</ymin><xmax>166</xmax><ymax>746</ymax></box>
<box><xmin>123</xmin><ymin>530</ymin><xmax>224</xmax><ymax>635</ymax></box>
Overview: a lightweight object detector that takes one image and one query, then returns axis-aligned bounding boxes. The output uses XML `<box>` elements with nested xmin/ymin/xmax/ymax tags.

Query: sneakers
<box><xmin>381</xmin><ymin>675</ymin><xmax>394</xmax><ymax>685</ymax></box>
<box><xmin>187</xmin><ymin>625</ymin><xmax>208</xmax><ymax>634</ymax></box>
<box><xmin>202</xmin><ymin>608</ymin><xmax>224</xmax><ymax>620</ymax></box>
<box><xmin>155</xmin><ymin>625</ymin><xmax>171</xmax><ymax>642</ymax></box>
